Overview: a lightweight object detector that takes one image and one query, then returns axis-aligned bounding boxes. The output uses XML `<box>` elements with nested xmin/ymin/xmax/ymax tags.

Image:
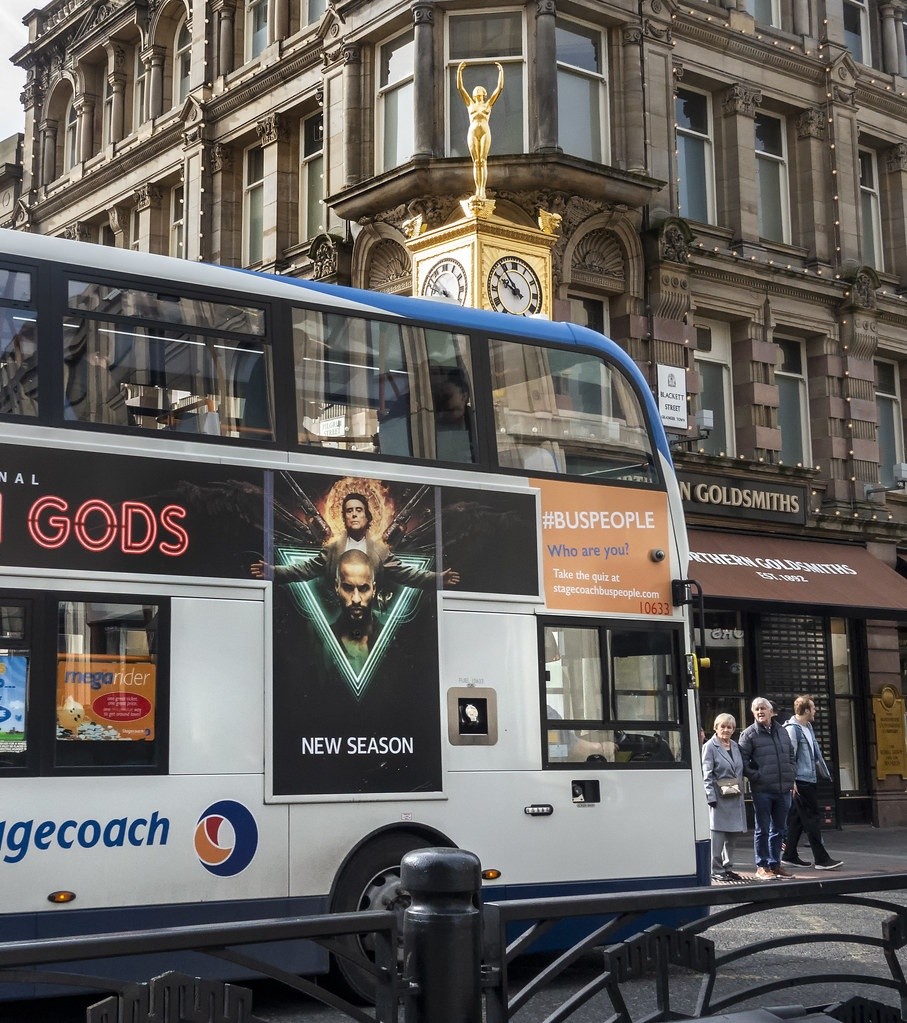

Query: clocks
<box><xmin>486</xmin><ymin>257</ymin><xmax>542</xmax><ymax>318</ymax></box>
<box><xmin>419</xmin><ymin>258</ymin><xmax>468</xmax><ymax>307</ymax></box>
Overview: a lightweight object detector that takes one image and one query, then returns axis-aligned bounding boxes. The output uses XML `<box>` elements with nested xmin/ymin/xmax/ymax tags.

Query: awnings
<box><xmin>682</xmin><ymin>528</ymin><xmax>907</xmax><ymax>613</ymax></box>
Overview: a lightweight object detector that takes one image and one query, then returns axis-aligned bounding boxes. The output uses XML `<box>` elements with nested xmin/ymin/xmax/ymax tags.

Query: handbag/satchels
<box><xmin>715</xmin><ymin>778</ymin><xmax>742</xmax><ymax>798</ymax></box>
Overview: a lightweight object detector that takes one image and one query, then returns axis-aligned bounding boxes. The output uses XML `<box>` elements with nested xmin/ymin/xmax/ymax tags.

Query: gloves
<box><xmin>708</xmin><ymin>801</ymin><xmax>716</xmax><ymax>808</ymax></box>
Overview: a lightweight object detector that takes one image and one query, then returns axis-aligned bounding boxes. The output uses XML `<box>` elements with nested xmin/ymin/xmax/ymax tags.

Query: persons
<box><xmin>737</xmin><ymin>695</ymin><xmax>797</xmax><ymax>882</ymax></box>
<box><xmin>326</xmin><ymin>550</ymin><xmax>387</xmax><ymax>673</ymax></box>
<box><xmin>457</xmin><ymin>59</ymin><xmax>506</xmax><ymax>199</ymax></box>
<box><xmin>547</xmin><ymin>704</ymin><xmax>618</xmax><ymax>764</ymax></box>
<box><xmin>701</xmin><ymin>712</ymin><xmax>748</xmax><ymax>884</ymax></box>
<box><xmin>243</xmin><ymin>346</ymin><xmax>330</xmax><ymax>445</ymax></box>
<box><xmin>777</xmin><ymin>695</ymin><xmax>845</xmax><ymax>873</ymax></box>
<box><xmin>370</xmin><ymin>365</ymin><xmax>483</xmax><ymax>468</ymax></box>
<box><xmin>249</xmin><ymin>493</ymin><xmax>460</xmax><ymax>600</ymax></box>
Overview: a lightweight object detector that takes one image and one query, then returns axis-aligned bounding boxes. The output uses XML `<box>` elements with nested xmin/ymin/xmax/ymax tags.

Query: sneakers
<box><xmin>755</xmin><ymin>866</ymin><xmax>776</xmax><ymax>880</ymax></box>
<box><xmin>712</xmin><ymin>872</ymin><xmax>733</xmax><ymax>881</ymax></box>
<box><xmin>770</xmin><ymin>868</ymin><xmax>796</xmax><ymax>879</ymax></box>
<box><xmin>781</xmin><ymin>856</ymin><xmax>812</xmax><ymax>868</ymax></box>
<box><xmin>815</xmin><ymin>859</ymin><xmax>844</xmax><ymax>869</ymax></box>
<box><xmin>726</xmin><ymin>871</ymin><xmax>741</xmax><ymax>879</ymax></box>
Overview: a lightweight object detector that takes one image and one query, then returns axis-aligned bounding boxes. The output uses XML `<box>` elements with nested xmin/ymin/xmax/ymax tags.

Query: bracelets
<box><xmin>600</xmin><ymin>742</ymin><xmax>605</xmax><ymax>757</ymax></box>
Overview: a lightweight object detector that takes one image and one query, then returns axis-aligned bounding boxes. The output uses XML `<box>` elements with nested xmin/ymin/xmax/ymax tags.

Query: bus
<box><xmin>0</xmin><ymin>220</ymin><xmax>732</xmax><ymax>1004</ymax></box>
